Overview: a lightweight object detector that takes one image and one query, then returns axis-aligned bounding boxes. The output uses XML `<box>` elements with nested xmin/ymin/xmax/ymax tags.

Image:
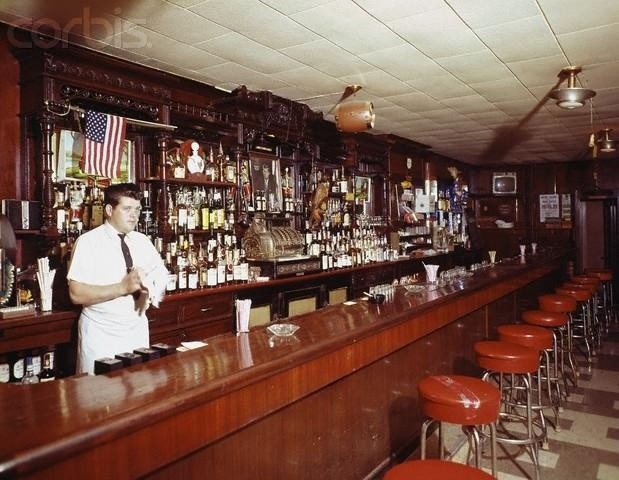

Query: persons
<box><xmin>254</xmin><ymin>164</ymin><xmax>276</xmax><ymax>192</ymax></box>
<box><xmin>66</xmin><ymin>183</ymin><xmax>168</xmax><ymax>375</ymax></box>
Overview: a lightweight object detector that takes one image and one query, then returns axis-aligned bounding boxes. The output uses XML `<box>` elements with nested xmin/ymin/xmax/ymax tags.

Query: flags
<box><xmin>85</xmin><ymin>110</ymin><xmax>126</xmax><ymax>179</ymax></box>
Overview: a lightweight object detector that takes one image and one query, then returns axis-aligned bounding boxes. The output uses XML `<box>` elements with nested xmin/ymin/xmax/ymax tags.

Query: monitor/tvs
<box><xmin>492</xmin><ymin>171</ymin><xmax>517</xmax><ymax>195</ymax></box>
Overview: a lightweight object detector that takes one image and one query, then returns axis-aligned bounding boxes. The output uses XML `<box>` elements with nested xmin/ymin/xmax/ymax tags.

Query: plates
<box><xmin>268</xmin><ymin>323</ymin><xmax>300</xmax><ymax>336</ymax></box>
<box><xmin>403</xmin><ymin>285</ymin><xmax>425</xmax><ymax>293</ymax></box>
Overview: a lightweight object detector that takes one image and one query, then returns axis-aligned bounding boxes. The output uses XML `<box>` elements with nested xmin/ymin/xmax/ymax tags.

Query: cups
<box><xmin>235</xmin><ymin>309</ymin><xmax>251</xmax><ymax>333</ymax></box>
<box><xmin>426</xmin><ymin>269</ymin><xmax>438</xmax><ymax>284</ymax></box>
<box><xmin>40</xmin><ymin>289</ymin><xmax>53</xmax><ymax>312</ymax></box>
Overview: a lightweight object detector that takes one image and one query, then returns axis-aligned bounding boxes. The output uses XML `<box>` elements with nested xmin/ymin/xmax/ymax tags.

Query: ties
<box><xmin>118</xmin><ymin>233</ymin><xmax>141</xmax><ymax>301</ymax></box>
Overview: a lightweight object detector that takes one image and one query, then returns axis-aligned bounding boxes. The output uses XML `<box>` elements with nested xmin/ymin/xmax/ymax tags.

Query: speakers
<box><xmin>1</xmin><ymin>199</ymin><xmax>23</xmax><ymax>231</ymax></box>
<box><xmin>29</xmin><ymin>199</ymin><xmax>43</xmax><ymax>231</ymax></box>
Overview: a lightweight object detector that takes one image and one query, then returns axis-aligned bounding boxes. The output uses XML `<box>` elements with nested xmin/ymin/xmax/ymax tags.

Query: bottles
<box><xmin>305</xmin><ymin>221</ymin><xmax>394</xmax><ymax>272</ymax></box>
<box><xmin>328</xmin><ymin>200</ymin><xmax>351</xmax><ymax>225</ymax></box>
<box><xmin>53</xmin><ymin>179</ymin><xmax>156</xmax><ymax>235</ymax></box>
<box><xmin>168</xmin><ymin>143</ymin><xmax>240</xmax><ymax>183</ymax></box>
<box><xmin>261</xmin><ymin>189</ymin><xmax>267</xmax><ymax>212</ymax></box>
<box><xmin>282</xmin><ymin>186</ymin><xmax>289</xmax><ymax>211</ymax></box>
<box><xmin>167</xmin><ymin>183</ymin><xmax>236</xmax><ymax>229</ymax></box>
<box><xmin>398</xmin><ymin>227</ymin><xmax>433</xmax><ymax>244</ymax></box>
<box><xmin>154</xmin><ymin>233</ymin><xmax>248</xmax><ymax>292</ymax></box>
<box><xmin>0</xmin><ymin>351</ymin><xmax>54</xmax><ymax>386</ymax></box>
<box><xmin>255</xmin><ymin>190</ymin><xmax>262</xmax><ymax>211</ymax></box>
<box><xmin>289</xmin><ymin>186</ymin><xmax>295</xmax><ymax>212</ymax></box>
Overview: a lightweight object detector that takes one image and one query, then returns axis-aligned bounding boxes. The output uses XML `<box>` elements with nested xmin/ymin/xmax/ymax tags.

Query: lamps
<box><xmin>596</xmin><ymin>127</ymin><xmax>619</xmax><ymax>152</ymax></box>
<box><xmin>551</xmin><ymin>63</ymin><xmax>596</xmax><ymax>114</ymax></box>
